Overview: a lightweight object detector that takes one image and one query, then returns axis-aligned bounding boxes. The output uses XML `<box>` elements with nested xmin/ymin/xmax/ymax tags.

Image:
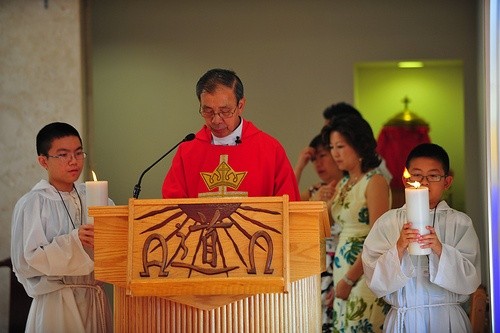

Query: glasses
<box><xmin>409</xmin><ymin>173</ymin><xmax>448</xmax><ymax>183</ymax></box>
<box><xmin>198</xmin><ymin>102</ymin><xmax>239</xmax><ymax>118</ymax></box>
<box><xmin>41</xmin><ymin>151</ymin><xmax>89</xmax><ymax>162</ymax></box>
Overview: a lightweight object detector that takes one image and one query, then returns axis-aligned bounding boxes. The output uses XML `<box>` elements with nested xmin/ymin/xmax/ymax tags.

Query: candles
<box><xmin>85</xmin><ymin>170</ymin><xmax>108</xmax><ymax>225</ymax></box>
<box><xmin>405</xmin><ymin>181</ymin><xmax>431</xmax><ymax>255</ymax></box>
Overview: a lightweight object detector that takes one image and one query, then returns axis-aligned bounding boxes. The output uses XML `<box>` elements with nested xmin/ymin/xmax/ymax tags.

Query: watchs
<box><xmin>343</xmin><ymin>274</ymin><xmax>356</xmax><ymax>287</ymax></box>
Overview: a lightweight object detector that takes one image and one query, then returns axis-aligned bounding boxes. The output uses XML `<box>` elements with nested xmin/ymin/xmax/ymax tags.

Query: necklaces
<box><xmin>422</xmin><ymin>200</ymin><xmax>443</xmax><ymax>262</ymax></box>
<box><xmin>47</xmin><ymin>180</ymin><xmax>83</xmax><ymax>231</ymax></box>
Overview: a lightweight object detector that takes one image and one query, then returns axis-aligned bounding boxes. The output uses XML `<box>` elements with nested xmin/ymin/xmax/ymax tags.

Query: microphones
<box><xmin>133</xmin><ymin>133</ymin><xmax>195</xmax><ymax>198</ymax></box>
<box><xmin>235</xmin><ymin>136</ymin><xmax>242</xmax><ymax>143</ymax></box>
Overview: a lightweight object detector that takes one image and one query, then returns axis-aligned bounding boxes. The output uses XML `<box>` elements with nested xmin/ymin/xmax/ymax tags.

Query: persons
<box><xmin>291</xmin><ymin>134</ymin><xmax>349</xmax><ymax>333</ymax></box>
<box><xmin>9</xmin><ymin>122</ymin><xmax>118</xmax><ymax>333</ymax></box>
<box><xmin>361</xmin><ymin>143</ymin><xmax>482</xmax><ymax>333</ymax></box>
<box><xmin>326</xmin><ymin>115</ymin><xmax>393</xmax><ymax>332</ymax></box>
<box><xmin>323</xmin><ymin>101</ymin><xmax>392</xmax><ymax>183</ymax></box>
<box><xmin>161</xmin><ymin>68</ymin><xmax>301</xmax><ymax>202</ymax></box>
